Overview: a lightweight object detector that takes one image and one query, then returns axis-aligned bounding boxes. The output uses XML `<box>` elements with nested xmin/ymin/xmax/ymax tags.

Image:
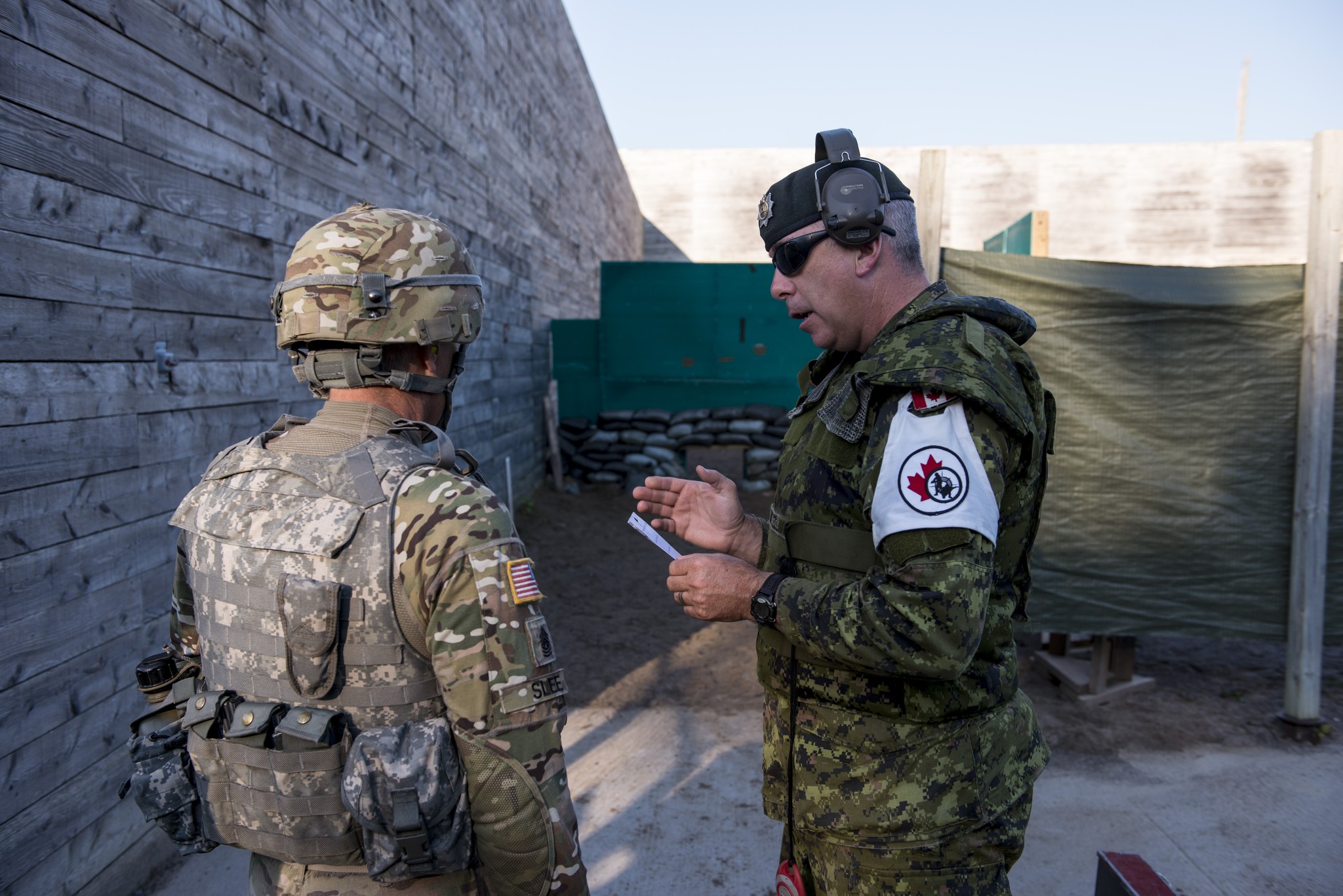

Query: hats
<box><xmin>759</xmin><ymin>157</ymin><xmax>915</xmax><ymax>253</ymax></box>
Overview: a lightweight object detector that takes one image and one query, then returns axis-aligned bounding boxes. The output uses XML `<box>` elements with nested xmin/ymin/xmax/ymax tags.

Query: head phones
<box><xmin>813</xmin><ymin>125</ymin><xmax>892</xmax><ymax>244</ymax></box>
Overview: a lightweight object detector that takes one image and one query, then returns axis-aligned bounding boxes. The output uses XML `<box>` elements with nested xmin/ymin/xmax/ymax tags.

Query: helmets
<box><xmin>275</xmin><ymin>203</ymin><xmax>485</xmax><ymax>352</ymax></box>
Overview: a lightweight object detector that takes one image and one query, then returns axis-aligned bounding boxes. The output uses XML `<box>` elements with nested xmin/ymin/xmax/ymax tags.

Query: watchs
<box><xmin>750</xmin><ymin>572</ymin><xmax>785</xmax><ymax>631</ymax></box>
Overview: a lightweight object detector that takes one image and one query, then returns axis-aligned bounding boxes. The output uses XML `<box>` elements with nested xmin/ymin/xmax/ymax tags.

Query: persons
<box><xmin>121</xmin><ymin>199</ymin><xmax>595</xmax><ymax>895</ymax></box>
<box><xmin>634</xmin><ymin>125</ymin><xmax>1064</xmax><ymax>896</ymax></box>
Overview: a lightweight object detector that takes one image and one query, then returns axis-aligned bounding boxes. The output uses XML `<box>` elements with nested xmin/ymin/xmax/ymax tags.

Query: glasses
<box><xmin>771</xmin><ymin>224</ymin><xmax>898</xmax><ymax>277</ymax></box>
